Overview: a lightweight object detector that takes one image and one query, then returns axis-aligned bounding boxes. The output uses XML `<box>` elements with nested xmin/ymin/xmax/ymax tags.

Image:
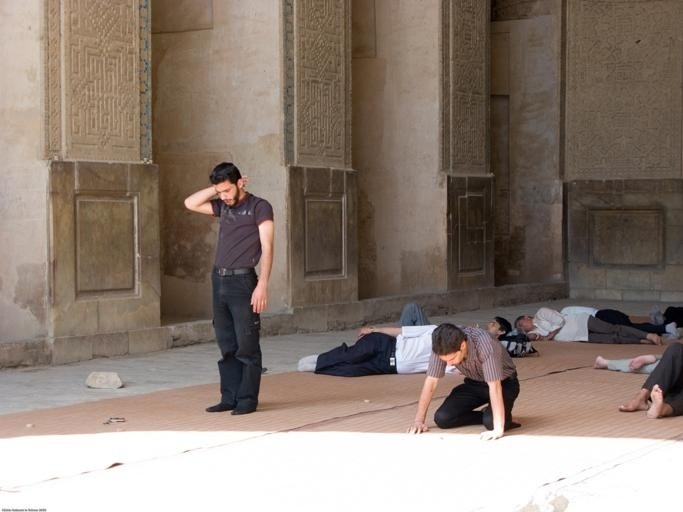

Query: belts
<box><xmin>218</xmin><ymin>268</ymin><xmax>248</xmax><ymax>276</ymax></box>
<box><xmin>390</xmin><ymin>343</ymin><xmax>396</xmax><ymax>372</ymax></box>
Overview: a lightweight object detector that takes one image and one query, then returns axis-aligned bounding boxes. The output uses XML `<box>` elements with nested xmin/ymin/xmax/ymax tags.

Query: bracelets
<box><xmin>369</xmin><ymin>326</ymin><xmax>374</xmax><ymax>332</ymax></box>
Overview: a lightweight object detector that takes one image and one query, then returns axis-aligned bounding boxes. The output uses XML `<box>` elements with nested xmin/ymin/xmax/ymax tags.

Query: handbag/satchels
<box><xmin>499</xmin><ymin>328</ymin><xmax>539</xmax><ymax>357</ymax></box>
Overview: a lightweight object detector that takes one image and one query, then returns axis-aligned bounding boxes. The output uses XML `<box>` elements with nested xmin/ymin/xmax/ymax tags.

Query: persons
<box><xmin>515</xmin><ymin>307</ymin><xmax>661</xmax><ymax>346</ymax></box>
<box><xmin>297</xmin><ymin>325</ymin><xmax>459</xmax><ymax>377</ymax></box>
<box><xmin>183</xmin><ymin>162</ymin><xmax>274</xmax><ymax>415</ymax></box>
<box><xmin>618</xmin><ymin>343</ymin><xmax>683</xmax><ymax>419</ymax></box>
<box><xmin>594</xmin><ymin>354</ymin><xmax>662</xmax><ymax>373</ymax></box>
<box><xmin>408</xmin><ymin>321</ymin><xmax>521</xmax><ymax>441</ymax></box>
<box><xmin>401</xmin><ymin>302</ymin><xmax>512</xmax><ymax>341</ymax></box>
<box><xmin>560</xmin><ymin>306</ymin><xmax>683</xmax><ymax>340</ymax></box>
